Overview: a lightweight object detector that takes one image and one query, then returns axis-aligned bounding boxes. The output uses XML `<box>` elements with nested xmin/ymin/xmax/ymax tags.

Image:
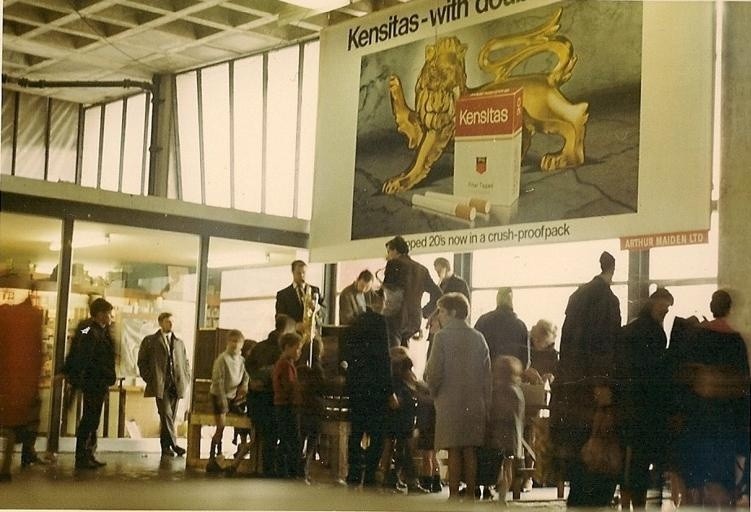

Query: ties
<box><xmin>166</xmin><ymin>335</ymin><xmax>170</xmax><ymax>345</ymax></box>
<box><xmin>298</xmin><ymin>286</ymin><xmax>303</xmax><ymax>296</ymax></box>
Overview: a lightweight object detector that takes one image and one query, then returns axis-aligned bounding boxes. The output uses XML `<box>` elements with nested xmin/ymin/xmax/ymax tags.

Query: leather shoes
<box><xmin>174</xmin><ymin>445</ymin><xmax>185</xmax><ymax>455</ymax></box>
<box><xmin>162</xmin><ymin>448</ymin><xmax>174</xmax><ymax>456</ymax></box>
<box><xmin>484</xmin><ymin>490</ymin><xmax>493</xmax><ymax>499</ymax></box>
<box><xmin>408</xmin><ymin>485</ymin><xmax>429</xmax><ymax>495</ymax></box>
<box><xmin>432</xmin><ymin>484</ymin><xmax>441</xmax><ymax>492</ymax></box>
<box><xmin>460</xmin><ymin>488</ymin><xmax>481</xmax><ymax>498</ymax></box>
<box><xmin>75</xmin><ymin>460</ymin><xmax>98</xmax><ymax>470</ymax></box>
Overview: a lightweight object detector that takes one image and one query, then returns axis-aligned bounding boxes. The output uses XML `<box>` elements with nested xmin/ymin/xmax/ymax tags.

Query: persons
<box><xmin>13</xmin><ymin>394</ymin><xmax>49</xmax><ymax>469</ymax></box>
<box><xmin>206</xmin><ymin>233</ymin><xmax>750</xmax><ymax>510</ymax></box>
<box><xmin>137</xmin><ymin>312</ymin><xmax>190</xmax><ymax>458</ymax></box>
<box><xmin>64</xmin><ymin>298</ymin><xmax>116</xmax><ymax>468</ymax></box>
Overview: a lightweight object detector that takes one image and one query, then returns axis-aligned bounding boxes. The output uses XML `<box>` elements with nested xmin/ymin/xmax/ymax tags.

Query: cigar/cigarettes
<box><xmin>411</xmin><ymin>193</ymin><xmax>476</xmax><ymax>220</ymax></box>
<box><xmin>424</xmin><ymin>190</ymin><xmax>490</xmax><ymax>213</ymax></box>
